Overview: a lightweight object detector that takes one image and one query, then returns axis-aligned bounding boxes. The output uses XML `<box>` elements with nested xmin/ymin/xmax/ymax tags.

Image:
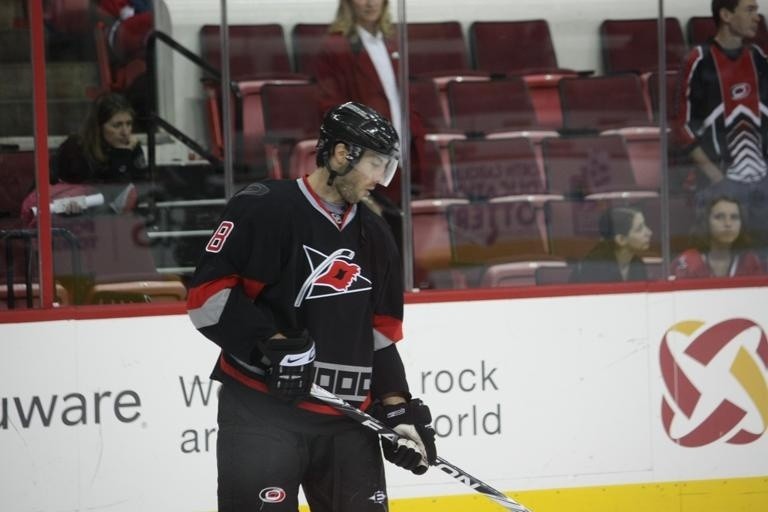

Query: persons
<box><xmin>26</xmin><ymin>91</ymin><xmax>148</xmax><ymax>199</ymax></box>
<box><xmin>188</xmin><ymin>102</ymin><xmax>438</xmax><ymax>512</ymax></box>
<box><xmin>306</xmin><ymin>0</ymin><xmax>439</xmax><ymax>209</ymax></box>
<box><xmin>576</xmin><ymin>205</ymin><xmax>653</xmax><ymax>283</ymax></box>
<box><xmin>670</xmin><ymin>194</ymin><xmax>762</xmax><ymax>278</ymax></box>
<box><xmin>671</xmin><ymin>0</ymin><xmax>768</xmax><ymax>197</ymax></box>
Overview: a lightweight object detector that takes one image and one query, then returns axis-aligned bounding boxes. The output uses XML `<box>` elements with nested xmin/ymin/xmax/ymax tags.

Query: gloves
<box><xmin>373</xmin><ymin>399</ymin><xmax>437</xmax><ymax>475</ymax></box>
<box><xmin>255</xmin><ymin>328</ymin><xmax>317</xmax><ymax>409</ymax></box>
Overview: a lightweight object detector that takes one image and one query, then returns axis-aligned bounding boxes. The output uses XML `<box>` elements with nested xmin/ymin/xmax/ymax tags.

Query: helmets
<box><xmin>315</xmin><ymin>100</ymin><xmax>401</xmax><ymax>189</ymax></box>
